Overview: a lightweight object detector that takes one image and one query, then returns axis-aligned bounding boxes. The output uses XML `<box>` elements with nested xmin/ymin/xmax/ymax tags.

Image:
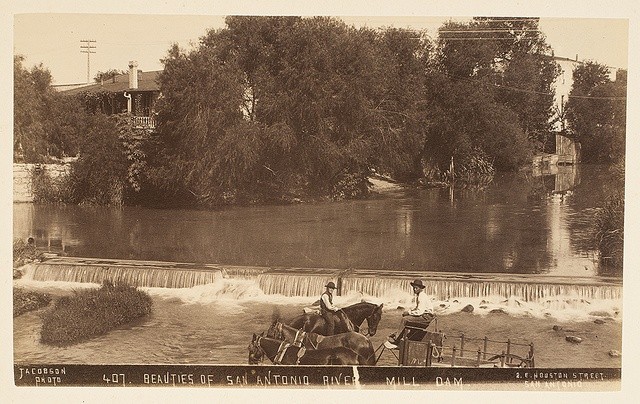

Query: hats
<box><xmin>325</xmin><ymin>282</ymin><xmax>337</xmax><ymax>289</ymax></box>
<box><xmin>410</xmin><ymin>280</ymin><xmax>425</xmax><ymax>289</ymax></box>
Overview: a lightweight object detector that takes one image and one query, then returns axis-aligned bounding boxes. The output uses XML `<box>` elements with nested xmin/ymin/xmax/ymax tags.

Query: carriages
<box><xmin>247</xmin><ymin>316</ymin><xmax>537</xmax><ymax>369</ymax></box>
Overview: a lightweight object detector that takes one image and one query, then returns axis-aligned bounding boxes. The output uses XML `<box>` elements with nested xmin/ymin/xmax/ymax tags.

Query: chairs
<box><xmin>374</xmin><ymin>314</ymin><xmax>437</xmax><ymax>366</ymax></box>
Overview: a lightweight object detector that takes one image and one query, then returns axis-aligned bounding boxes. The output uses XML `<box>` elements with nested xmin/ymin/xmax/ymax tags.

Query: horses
<box><xmin>267</xmin><ymin>315</ymin><xmax>375</xmax><ymax>365</ymax></box>
<box><xmin>248</xmin><ymin>331</ymin><xmax>364</xmax><ymax>365</ymax></box>
<box><xmin>290</xmin><ymin>301</ymin><xmax>384</xmax><ymax>337</ymax></box>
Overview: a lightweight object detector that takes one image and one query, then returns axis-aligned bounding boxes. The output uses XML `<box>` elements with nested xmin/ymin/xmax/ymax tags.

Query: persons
<box><xmin>385</xmin><ymin>279</ymin><xmax>434</xmax><ymax>343</ymax></box>
<box><xmin>320</xmin><ymin>282</ymin><xmax>341</xmax><ymax>336</ymax></box>
